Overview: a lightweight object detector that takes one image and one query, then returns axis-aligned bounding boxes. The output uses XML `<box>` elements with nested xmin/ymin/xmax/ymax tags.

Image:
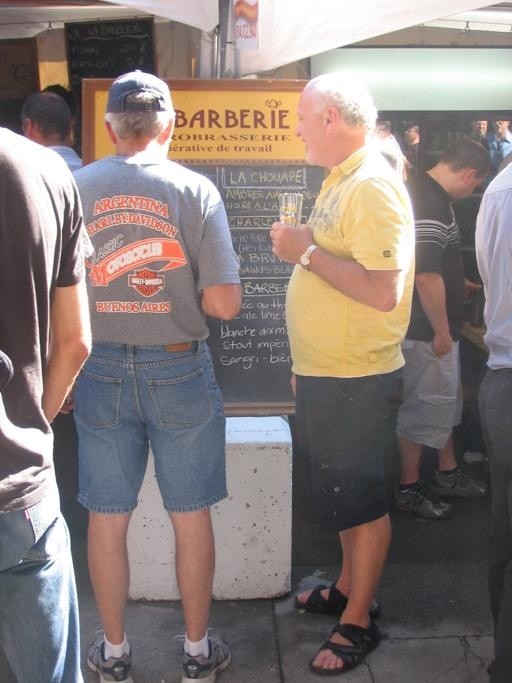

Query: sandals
<box><xmin>307</xmin><ymin>616</ymin><xmax>383</xmax><ymax>678</ymax></box>
<box><xmin>292</xmin><ymin>577</ymin><xmax>383</xmax><ymax>619</ymax></box>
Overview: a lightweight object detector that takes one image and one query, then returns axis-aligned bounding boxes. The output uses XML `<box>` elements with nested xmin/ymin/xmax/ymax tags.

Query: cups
<box><xmin>277</xmin><ymin>191</ymin><xmax>304</xmax><ymax>228</ymax></box>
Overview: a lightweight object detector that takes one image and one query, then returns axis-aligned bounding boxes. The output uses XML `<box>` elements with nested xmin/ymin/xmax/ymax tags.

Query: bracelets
<box><xmin>300</xmin><ymin>244</ymin><xmax>320</xmax><ymax>270</ymax></box>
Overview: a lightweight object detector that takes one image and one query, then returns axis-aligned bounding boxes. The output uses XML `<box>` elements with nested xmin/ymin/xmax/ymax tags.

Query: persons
<box><xmin>402</xmin><ymin>138</ymin><xmax>488</xmax><ymax>521</ymax></box>
<box><xmin>57</xmin><ymin>66</ymin><xmax>244</xmax><ymax>681</ymax></box>
<box><xmin>381</xmin><ymin>109</ymin><xmax>512</xmax><ymax>189</ymax></box>
<box><xmin>472</xmin><ymin>161</ymin><xmax>512</xmax><ymax>681</ymax></box>
<box><xmin>1</xmin><ymin>125</ymin><xmax>96</xmax><ymax>680</ymax></box>
<box><xmin>40</xmin><ymin>84</ymin><xmax>83</xmax><ymax>160</ymax></box>
<box><xmin>15</xmin><ymin>90</ymin><xmax>87</xmax><ymax>174</ymax></box>
<box><xmin>268</xmin><ymin>69</ymin><xmax>417</xmax><ymax>677</ymax></box>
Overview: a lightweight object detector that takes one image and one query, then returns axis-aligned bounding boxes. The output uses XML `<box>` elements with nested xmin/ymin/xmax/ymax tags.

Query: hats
<box><xmin>104</xmin><ymin>68</ymin><xmax>178</xmax><ymax>113</ymax></box>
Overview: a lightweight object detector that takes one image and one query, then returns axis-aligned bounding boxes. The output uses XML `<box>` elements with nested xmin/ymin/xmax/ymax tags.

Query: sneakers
<box><xmin>179</xmin><ymin>633</ymin><xmax>234</xmax><ymax>683</ymax></box>
<box><xmin>426</xmin><ymin>462</ymin><xmax>493</xmax><ymax>499</ymax></box>
<box><xmin>84</xmin><ymin>633</ymin><xmax>136</xmax><ymax>683</ymax></box>
<box><xmin>389</xmin><ymin>481</ymin><xmax>456</xmax><ymax>523</ymax></box>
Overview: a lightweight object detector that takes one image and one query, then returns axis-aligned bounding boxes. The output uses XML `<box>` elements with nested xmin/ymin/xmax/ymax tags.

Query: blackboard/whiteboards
<box><xmin>179</xmin><ymin>165</ymin><xmax>324</xmax><ymax>417</ymax></box>
<box><xmin>64</xmin><ymin>17</ymin><xmax>158</xmax><ymax>157</ymax></box>
